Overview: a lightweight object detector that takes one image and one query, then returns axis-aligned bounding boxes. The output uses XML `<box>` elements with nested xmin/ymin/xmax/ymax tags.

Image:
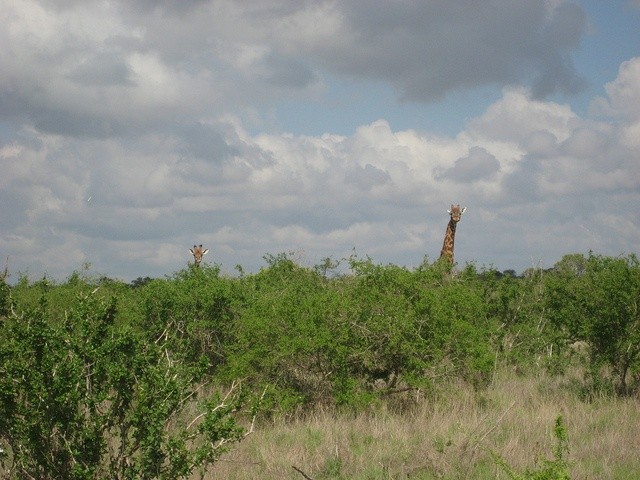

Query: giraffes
<box><xmin>188</xmin><ymin>245</ymin><xmax>209</xmax><ymax>268</ymax></box>
<box><xmin>431</xmin><ymin>203</ymin><xmax>467</xmax><ymax>272</ymax></box>
